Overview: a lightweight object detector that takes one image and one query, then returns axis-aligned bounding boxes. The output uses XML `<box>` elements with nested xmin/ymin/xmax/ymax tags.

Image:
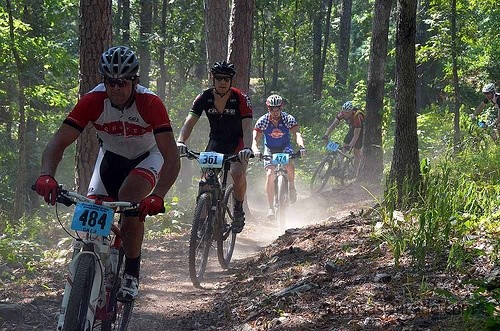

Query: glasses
<box><xmin>107</xmin><ymin>79</ymin><xmax>128</xmax><ymax>87</ymax></box>
<box><xmin>215</xmin><ymin>76</ymin><xmax>232</xmax><ymax>82</ymax></box>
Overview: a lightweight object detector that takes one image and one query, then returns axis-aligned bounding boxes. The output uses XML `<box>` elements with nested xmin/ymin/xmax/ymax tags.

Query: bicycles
<box><xmin>32</xmin><ymin>181</ymin><xmax>163</xmax><ymax>331</ymax></box>
<box><xmin>309</xmin><ymin>133</ymin><xmax>359</xmax><ymax>197</ymax></box>
<box><xmin>176</xmin><ymin>147</ymin><xmax>263</xmax><ymax>287</ymax></box>
<box><xmin>252</xmin><ymin>151</ymin><xmax>301</xmax><ymax>236</ymax></box>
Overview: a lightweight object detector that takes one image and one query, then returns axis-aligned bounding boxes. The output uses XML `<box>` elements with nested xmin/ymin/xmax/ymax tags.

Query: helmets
<box><xmin>482</xmin><ymin>83</ymin><xmax>496</xmax><ymax>94</ymax></box>
<box><xmin>211</xmin><ymin>60</ymin><xmax>236</xmax><ymax>77</ymax></box>
<box><xmin>342</xmin><ymin>101</ymin><xmax>354</xmax><ymax>112</ymax></box>
<box><xmin>98</xmin><ymin>46</ymin><xmax>140</xmax><ymax>81</ymax></box>
<box><xmin>266</xmin><ymin>94</ymin><xmax>284</xmax><ymax>107</ymax></box>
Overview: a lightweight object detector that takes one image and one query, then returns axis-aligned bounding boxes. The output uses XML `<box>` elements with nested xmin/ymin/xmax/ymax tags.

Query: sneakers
<box><xmin>116</xmin><ymin>273</ymin><xmax>139</xmax><ymax>303</ymax></box>
<box><xmin>231</xmin><ymin>208</ymin><xmax>245</xmax><ymax>233</ymax></box>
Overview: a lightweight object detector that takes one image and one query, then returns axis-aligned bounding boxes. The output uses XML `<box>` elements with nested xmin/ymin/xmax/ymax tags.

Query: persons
<box><xmin>176</xmin><ymin>60</ymin><xmax>253</xmax><ymax>240</ymax></box>
<box><xmin>252</xmin><ymin>94</ymin><xmax>306</xmax><ymax>219</ymax></box>
<box><xmin>472</xmin><ymin>84</ymin><xmax>500</xmax><ymax>143</ymax></box>
<box><xmin>323</xmin><ymin>102</ymin><xmax>363</xmax><ymax>179</ymax></box>
<box><xmin>35</xmin><ymin>46</ymin><xmax>181</xmax><ymax>318</ymax></box>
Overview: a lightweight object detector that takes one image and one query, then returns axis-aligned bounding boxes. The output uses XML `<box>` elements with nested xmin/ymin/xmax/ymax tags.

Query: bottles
<box><xmin>110</xmin><ymin>244</ymin><xmax>119</xmax><ymax>273</ymax></box>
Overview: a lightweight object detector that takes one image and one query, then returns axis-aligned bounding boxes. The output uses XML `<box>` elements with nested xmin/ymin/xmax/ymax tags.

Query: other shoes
<box><xmin>269</xmin><ymin>209</ymin><xmax>275</xmax><ymax>216</ymax></box>
<box><xmin>290</xmin><ymin>192</ymin><xmax>295</xmax><ymax>201</ymax></box>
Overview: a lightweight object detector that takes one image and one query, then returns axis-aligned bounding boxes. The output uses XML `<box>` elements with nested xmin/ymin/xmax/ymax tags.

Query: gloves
<box><xmin>254</xmin><ymin>150</ymin><xmax>261</xmax><ymax>159</ymax></box>
<box><xmin>34</xmin><ymin>175</ymin><xmax>58</xmax><ymax>205</ymax></box>
<box><xmin>177</xmin><ymin>142</ymin><xmax>186</xmax><ymax>154</ymax></box>
<box><xmin>344</xmin><ymin>144</ymin><xmax>352</xmax><ymax>150</ymax></box>
<box><xmin>298</xmin><ymin>148</ymin><xmax>306</xmax><ymax>158</ymax></box>
<box><xmin>238</xmin><ymin>148</ymin><xmax>252</xmax><ymax>161</ymax></box>
<box><xmin>137</xmin><ymin>194</ymin><xmax>164</xmax><ymax>222</ymax></box>
<box><xmin>321</xmin><ymin>135</ymin><xmax>328</xmax><ymax>140</ymax></box>
